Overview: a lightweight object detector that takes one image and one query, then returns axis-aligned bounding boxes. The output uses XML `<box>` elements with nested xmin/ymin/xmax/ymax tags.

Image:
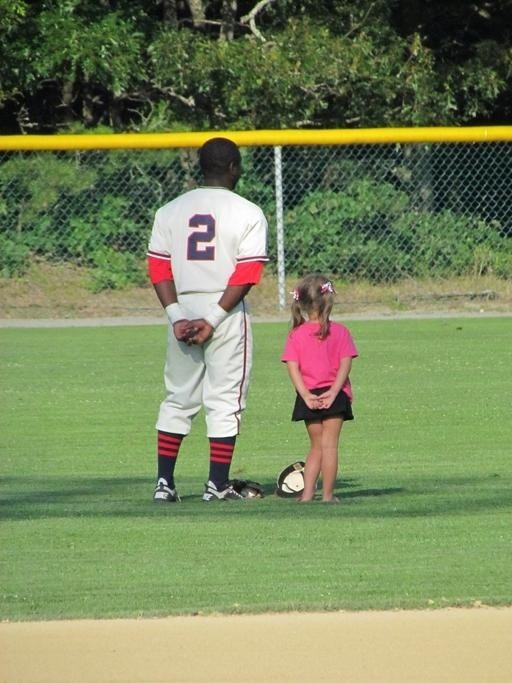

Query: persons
<box><xmin>280</xmin><ymin>273</ymin><xmax>359</xmax><ymax>504</ymax></box>
<box><xmin>144</xmin><ymin>135</ymin><xmax>272</xmax><ymax>504</ymax></box>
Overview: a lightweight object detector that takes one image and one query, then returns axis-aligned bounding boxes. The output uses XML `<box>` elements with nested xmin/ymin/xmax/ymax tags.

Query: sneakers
<box><xmin>152</xmin><ymin>480</ymin><xmax>183</xmax><ymax>504</ymax></box>
<box><xmin>203</xmin><ymin>483</ymin><xmax>246</xmax><ymax>502</ymax></box>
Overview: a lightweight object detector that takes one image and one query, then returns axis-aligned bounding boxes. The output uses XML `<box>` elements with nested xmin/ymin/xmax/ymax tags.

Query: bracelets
<box><xmin>164</xmin><ymin>302</ymin><xmax>186</xmax><ymax>325</ymax></box>
<box><xmin>204</xmin><ymin>304</ymin><xmax>231</xmax><ymax>329</ymax></box>
